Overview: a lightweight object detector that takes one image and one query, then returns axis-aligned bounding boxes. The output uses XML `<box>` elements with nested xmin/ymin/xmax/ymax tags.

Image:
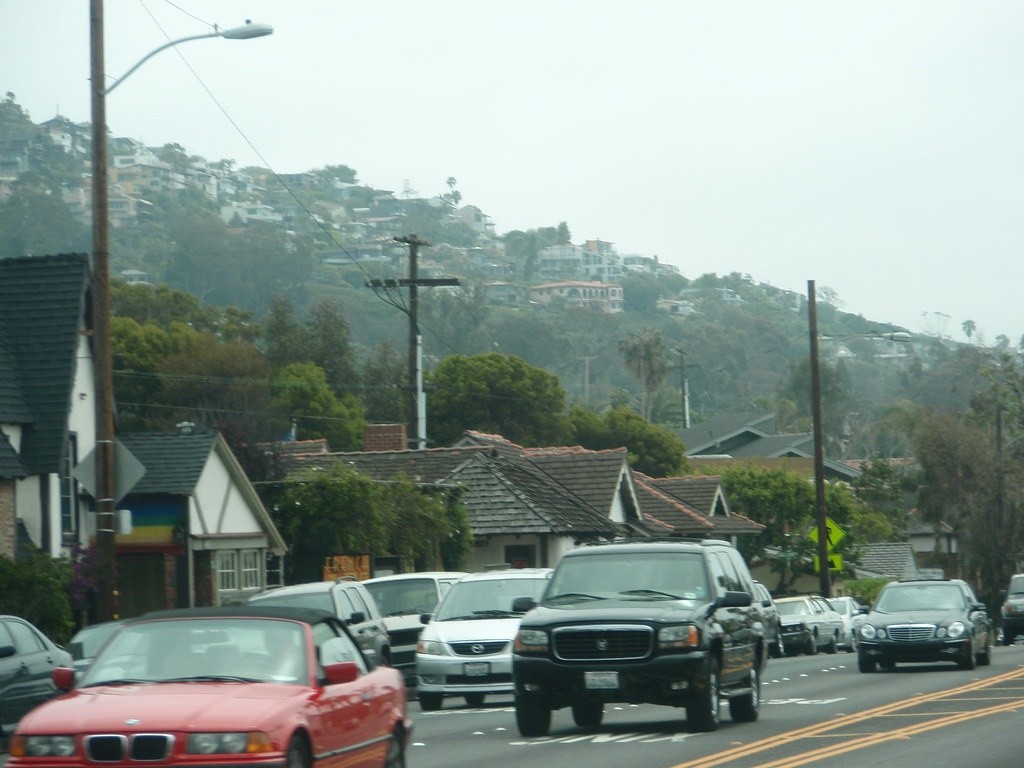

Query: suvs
<box><xmin>512</xmin><ymin>539</ymin><xmax>768</xmax><ymax>738</ymax></box>
<box><xmin>244</xmin><ymin>576</ymin><xmax>393</xmax><ymax>669</ymax></box>
<box><xmin>1000</xmin><ymin>574</ymin><xmax>1024</xmax><ymax>644</ymax></box>
<box><xmin>753</xmin><ymin>580</ymin><xmax>784</xmax><ymax>658</ymax></box>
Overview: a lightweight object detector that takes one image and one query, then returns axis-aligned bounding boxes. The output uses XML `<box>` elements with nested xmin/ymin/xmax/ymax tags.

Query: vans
<box><xmin>359</xmin><ymin>572</ymin><xmax>474</xmax><ymax>693</ymax></box>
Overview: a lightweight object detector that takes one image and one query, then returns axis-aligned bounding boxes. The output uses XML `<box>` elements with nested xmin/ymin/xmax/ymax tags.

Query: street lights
<box><xmin>808</xmin><ymin>280</ymin><xmax>911</xmax><ymax>594</ymax></box>
<box><xmin>89</xmin><ymin>0</ymin><xmax>275</xmax><ymax>621</ymax></box>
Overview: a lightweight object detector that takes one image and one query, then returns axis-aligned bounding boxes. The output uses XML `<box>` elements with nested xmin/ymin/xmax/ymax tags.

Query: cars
<box><xmin>858</xmin><ymin>578</ymin><xmax>992</xmax><ymax>673</ymax></box>
<box><xmin>825</xmin><ymin>597</ymin><xmax>868</xmax><ymax>652</ymax></box>
<box><xmin>8</xmin><ymin>606</ymin><xmax>415</xmax><ymax>768</ymax></box>
<box><xmin>770</xmin><ymin>595</ymin><xmax>843</xmax><ymax>656</ymax></box>
<box><xmin>63</xmin><ymin>619</ymin><xmax>149</xmax><ymax>683</ymax></box>
<box><xmin>415</xmin><ymin>567</ymin><xmax>559</xmax><ymax>709</ymax></box>
<box><xmin>0</xmin><ymin>616</ymin><xmax>74</xmax><ymax>726</ymax></box>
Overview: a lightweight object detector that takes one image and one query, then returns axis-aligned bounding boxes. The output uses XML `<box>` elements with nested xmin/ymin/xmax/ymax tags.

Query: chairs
<box><xmin>193</xmin><ymin>644</ymin><xmax>246</xmax><ymax>678</ymax></box>
